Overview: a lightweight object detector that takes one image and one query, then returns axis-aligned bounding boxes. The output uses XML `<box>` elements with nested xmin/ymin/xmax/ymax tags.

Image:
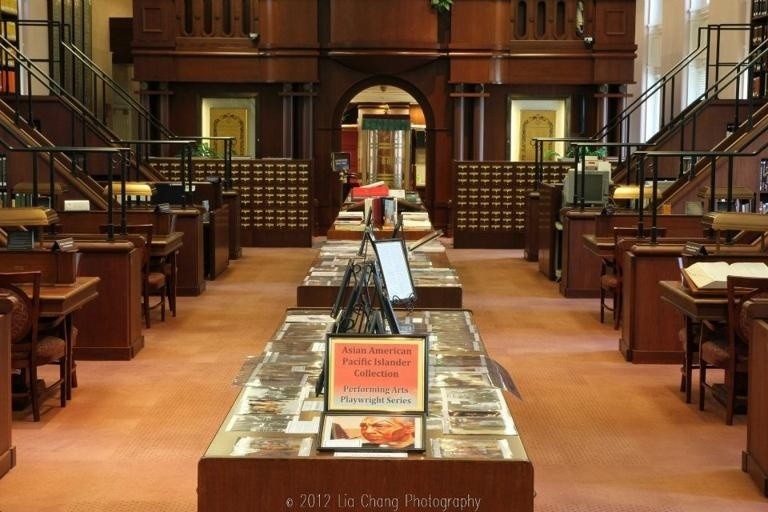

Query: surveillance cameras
<box><xmin>250</xmin><ymin>33</ymin><xmax>259</xmax><ymax>41</ymax></box>
<box><xmin>583</xmin><ymin>37</ymin><xmax>594</xmax><ymax>49</ymax></box>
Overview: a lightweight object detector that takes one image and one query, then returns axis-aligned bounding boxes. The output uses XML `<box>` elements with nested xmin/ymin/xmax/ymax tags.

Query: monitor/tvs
<box><xmin>564</xmin><ymin>168</ymin><xmax>611</xmax><ymax>207</ymax></box>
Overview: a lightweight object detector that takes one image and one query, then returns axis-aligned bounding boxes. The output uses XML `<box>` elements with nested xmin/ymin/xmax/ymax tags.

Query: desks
<box><xmin>579</xmin><ymin>232</ymin><xmax>739</xmax><ymax>406</ymax></box>
<box><xmin>7</xmin><ymin>208</ymin><xmax>207</xmax><ymax>396</ymax></box>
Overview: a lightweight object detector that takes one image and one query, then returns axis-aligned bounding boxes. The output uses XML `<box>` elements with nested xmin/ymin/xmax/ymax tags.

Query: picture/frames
<box><xmin>315</xmin><ymin>331</ymin><xmax>430</xmax><ymax>453</ymax></box>
<box><xmin>504</xmin><ymin>91</ymin><xmax>574</xmax><ymax>161</ymax></box>
<box><xmin>195</xmin><ymin>90</ymin><xmax>262</xmax><ymax>158</ymax></box>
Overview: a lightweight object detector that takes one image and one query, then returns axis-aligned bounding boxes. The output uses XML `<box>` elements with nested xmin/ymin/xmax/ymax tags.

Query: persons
<box><xmin>356</xmin><ymin>416</ymin><xmax>415</xmax><ymax>450</ymax></box>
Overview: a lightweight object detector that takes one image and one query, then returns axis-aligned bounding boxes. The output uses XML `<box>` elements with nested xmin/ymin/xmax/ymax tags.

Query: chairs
<box><xmin>599</xmin><ymin>226</ymin><xmax>667</xmax><ymax>330</ymax></box>
<box><xmin>698</xmin><ymin>274</ymin><xmax>768</xmax><ymax>426</ymax></box>
<box><xmin>0</xmin><ymin>269</ymin><xmax>70</xmax><ymax>423</ymax></box>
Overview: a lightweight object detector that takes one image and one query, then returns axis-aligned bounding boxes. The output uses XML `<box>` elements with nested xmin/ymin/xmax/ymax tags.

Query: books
<box><xmin>333</xmin><ymin>179</ymin><xmax>433</xmax><ymax>230</ymax></box>
<box><xmin>684</xmin><ymin>259</ymin><xmax>768</xmax><ymax>290</ymax></box>
